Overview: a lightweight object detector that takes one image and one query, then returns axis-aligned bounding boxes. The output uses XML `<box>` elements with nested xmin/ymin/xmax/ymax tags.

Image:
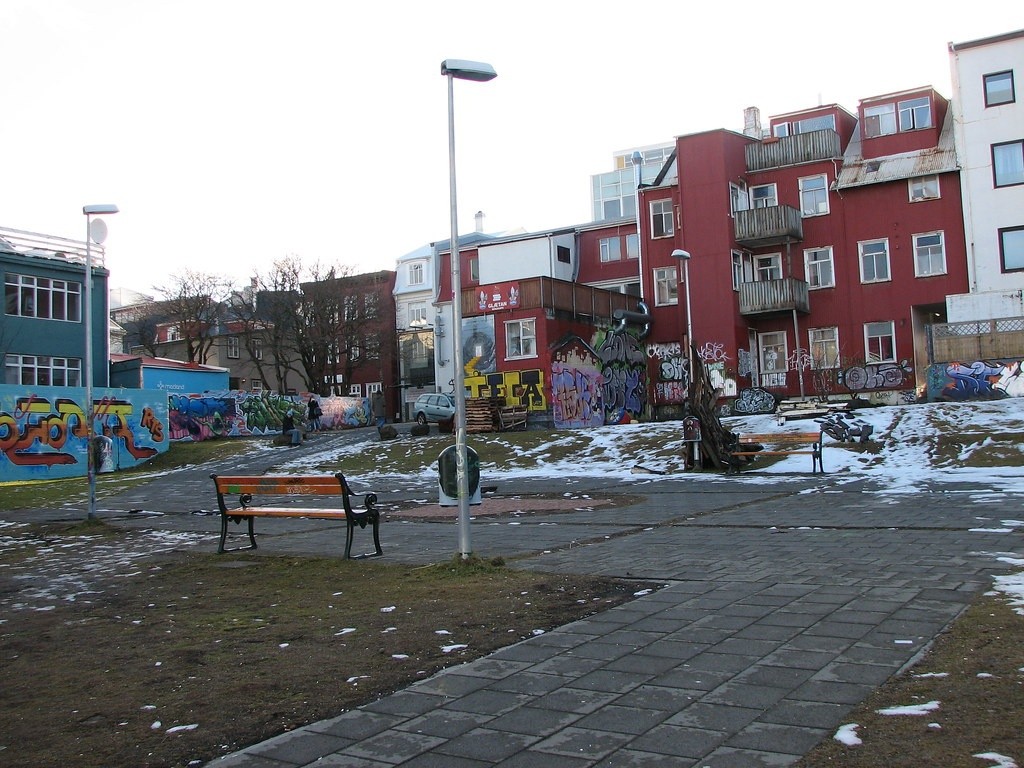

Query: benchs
<box><xmin>731</xmin><ymin>430</ymin><xmax>825</xmax><ymax>474</ymax></box>
<box><xmin>209</xmin><ymin>473</ymin><xmax>381</xmax><ymax>560</ymax></box>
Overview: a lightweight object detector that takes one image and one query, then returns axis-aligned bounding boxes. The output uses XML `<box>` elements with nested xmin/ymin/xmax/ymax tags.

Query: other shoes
<box><xmin>311</xmin><ymin>429</ymin><xmax>320</xmax><ymax>432</ymax></box>
<box><xmin>291</xmin><ymin>443</ymin><xmax>302</xmax><ymax>446</ymax></box>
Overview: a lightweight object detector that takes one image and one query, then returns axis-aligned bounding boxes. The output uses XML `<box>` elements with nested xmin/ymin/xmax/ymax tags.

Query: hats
<box><xmin>310</xmin><ymin>395</ymin><xmax>314</xmax><ymax>398</ymax></box>
<box><xmin>287</xmin><ymin>410</ymin><xmax>292</xmax><ymax>414</ymax></box>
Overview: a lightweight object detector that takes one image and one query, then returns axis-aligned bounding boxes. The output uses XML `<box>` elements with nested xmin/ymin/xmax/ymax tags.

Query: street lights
<box><xmin>671</xmin><ymin>249</ymin><xmax>700</xmax><ymax>465</ymax></box>
<box><xmin>82</xmin><ymin>202</ymin><xmax>121</xmax><ymax>518</ymax></box>
<box><xmin>442</xmin><ymin>57</ymin><xmax>497</xmax><ymax>558</ymax></box>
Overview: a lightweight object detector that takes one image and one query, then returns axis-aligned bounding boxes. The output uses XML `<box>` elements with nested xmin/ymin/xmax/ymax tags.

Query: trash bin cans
<box><xmin>93</xmin><ymin>435</ymin><xmax>115</xmax><ymax>474</ymax></box>
<box><xmin>436</xmin><ymin>443</ymin><xmax>483</xmax><ymax>507</ymax></box>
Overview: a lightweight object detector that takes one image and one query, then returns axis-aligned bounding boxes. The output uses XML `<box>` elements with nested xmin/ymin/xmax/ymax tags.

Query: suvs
<box><xmin>413</xmin><ymin>394</ymin><xmax>455</xmax><ymax>426</ymax></box>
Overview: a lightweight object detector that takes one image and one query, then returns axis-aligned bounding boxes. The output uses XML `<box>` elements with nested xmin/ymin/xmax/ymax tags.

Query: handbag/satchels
<box><xmin>314</xmin><ymin>407</ymin><xmax>323</xmax><ymax>416</ymax></box>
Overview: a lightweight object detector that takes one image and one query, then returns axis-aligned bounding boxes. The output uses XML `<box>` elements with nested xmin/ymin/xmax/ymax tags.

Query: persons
<box><xmin>372</xmin><ymin>390</ymin><xmax>385</xmax><ymax>432</ymax></box>
<box><xmin>282</xmin><ymin>410</ymin><xmax>302</xmax><ymax>447</ymax></box>
<box><xmin>307</xmin><ymin>396</ymin><xmax>323</xmax><ymax>432</ymax></box>
